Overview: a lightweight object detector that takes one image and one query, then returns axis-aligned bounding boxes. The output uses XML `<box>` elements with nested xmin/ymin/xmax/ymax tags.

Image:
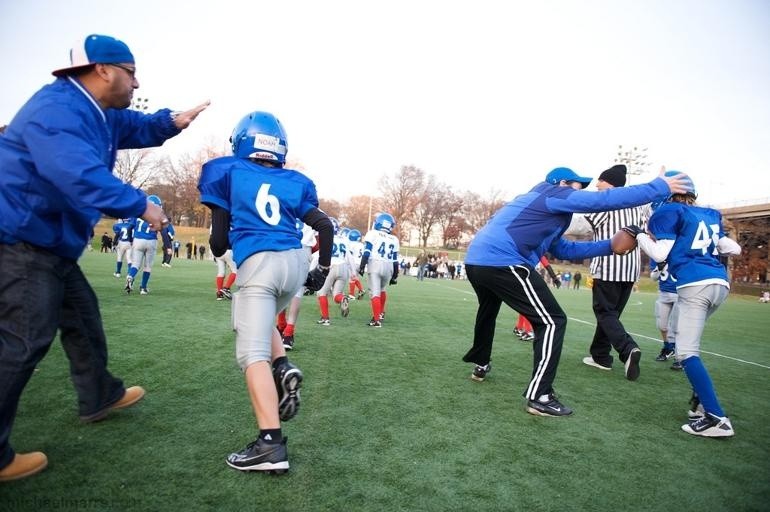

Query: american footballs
<box><xmin>611</xmin><ymin>230</ymin><xmax>637</xmax><ymax>255</ymax></box>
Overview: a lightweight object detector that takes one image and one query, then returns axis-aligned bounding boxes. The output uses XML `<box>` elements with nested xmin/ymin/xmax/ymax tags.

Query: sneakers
<box><xmin>623</xmin><ymin>345</ymin><xmax>641</xmax><ymax>381</ymax></box>
<box><xmin>0</xmin><ymin>448</ymin><xmax>49</xmax><ymax>486</ymax></box>
<box><xmin>511</xmin><ymin>325</ymin><xmax>523</xmax><ymax>337</ymax></box>
<box><xmin>471</xmin><ymin>360</ymin><xmax>492</xmax><ymax>382</ymax></box>
<box><xmin>224</xmin><ymin>436</ymin><xmax>291</xmax><ymax>477</ymax></box>
<box><xmin>688</xmin><ymin>393</ymin><xmax>706</xmax><ymax>420</ymax></box>
<box><xmin>112</xmin><ymin>260</ymin><xmax>386</xmax><ymax>350</ymax></box>
<box><xmin>581</xmin><ymin>356</ymin><xmax>612</xmax><ymax>371</ymax></box>
<box><xmin>655</xmin><ymin>341</ymin><xmax>676</xmax><ymax>362</ymax></box>
<box><xmin>680</xmin><ymin>411</ymin><xmax>735</xmax><ymax>438</ymax></box>
<box><xmin>669</xmin><ymin>361</ymin><xmax>682</xmax><ymax>371</ymax></box>
<box><xmin>518</xmin><ymin>333</ymin><xmax>535</xmax><ymax>342</ymax></box>
<box><xmin>273</xmin><ymin>356</ymin><xmax>306</xmax><ymax>424</ymax></box>
<box><xmin>525</xmin><ymin>393</ymin><xmax>573</xmax><ymax>418</ymax></box>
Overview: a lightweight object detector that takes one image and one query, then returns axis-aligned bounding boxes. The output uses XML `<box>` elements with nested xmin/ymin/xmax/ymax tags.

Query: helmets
<box><xmin>340</xmin><ymin>227</ymin><xmax>351</xmax><ymax>238</ymax></box>
<box><xmin>373</xmin><ymin>211</ymin><xmax>396</xmax><ymax>235</ymax></box>
<box><xmin>649</xmin><ymin>169</ymin><xmax>696</xmax><ymax>211</ymax></box>
<box><xmin>227</xmin><ymin>106</ymin><xmax>290</xmax><ymax>166</ymax></box>
<box><xmin>326</xmin><ymin>216</ymin><xmax>340</xmax><ymax>235</ymax></box>
<box><xmin>348</xmin><ymin>228</ymin><xmax>363</xmax><ymax>242</ymax></box>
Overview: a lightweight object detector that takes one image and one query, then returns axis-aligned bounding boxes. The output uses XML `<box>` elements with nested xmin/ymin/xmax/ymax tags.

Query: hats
<box><xmin>596</xmin><ymin>163</ymin><xmax>628</xmax><ymax>188</ymax></box>
<box><xmin>544</xmin><ymin>166</ymin><xmax>594</xmax><ymax>188</ymax></box>
<box><xmin>51</xmin><ymin>31</ymin><xmax>136</xmax><ymax>78</ymax></box>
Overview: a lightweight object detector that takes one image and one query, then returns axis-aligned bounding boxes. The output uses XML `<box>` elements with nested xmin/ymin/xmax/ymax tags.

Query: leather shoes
<box><xmin>77</xmin><ymin>380</ymin><xmax>146</xmax><ymax>425</ymax></box>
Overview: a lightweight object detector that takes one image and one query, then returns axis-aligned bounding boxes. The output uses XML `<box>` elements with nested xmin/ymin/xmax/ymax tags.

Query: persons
<box><xmin>621</xmin><ymin>170</ymin><xmax>742</xmax><ymax>438</ymax></box>
<box><xmin>0</xmin><ymin>32</ymin><xmax>213</xmax><ymax>481</ymax></box>
<box><xmin>103</xmin><ymin>197</ymin><xmax>237</xmax><ymax>300</ymax></box>
<box><xmin>649</xmin><ymin>258</ymin><xmax>686</xmax><ymax>371</ymax></box>
<box><xmin>542</xmin><ymin>268</ymin><xmax>580</xmax><ymax>289</ymax></box>
<box><xmin>195</xmin><ymin>108</ymin><xmax>335</xmax><ymax>477</ymax></box>
<box><xmin>563</xmin><ymin>163</ymin><xmax>653</xmax><ymax>380</ymax></box>
<box><xmin>462</xmin><ymin>167</ymin><xmax>693</xmax><ymax>419</ymax></box>
<box><xmin>304</xmin><ymin>212</ymin><xmax>467</xmax><ymax>326</ymax></box>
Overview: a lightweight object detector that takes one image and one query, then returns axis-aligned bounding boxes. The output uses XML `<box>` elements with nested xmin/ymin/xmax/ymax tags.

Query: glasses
<box><xmin>107</xmin><ymin>62</ymin><xmax>136</xmax><ymax>77</ymax></box>
